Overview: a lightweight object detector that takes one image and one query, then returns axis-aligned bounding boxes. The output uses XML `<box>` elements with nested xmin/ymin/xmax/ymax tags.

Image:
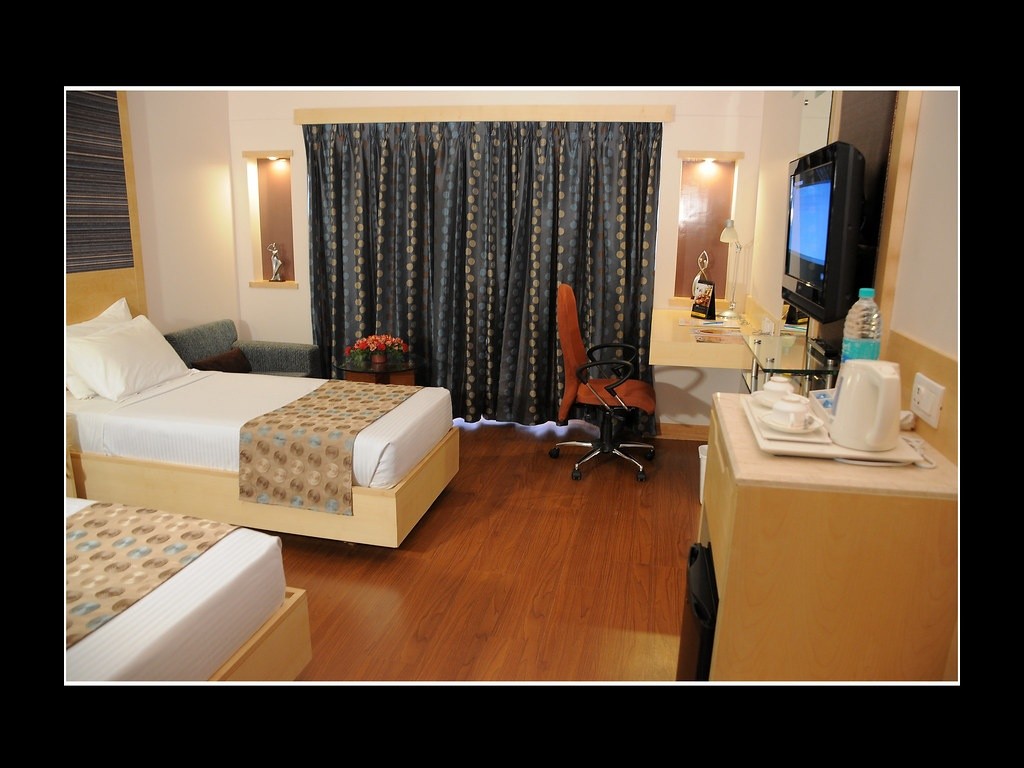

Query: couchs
<box><xmin>165</xmin><ymin>318</ymin><xmax>320</xmax><ymax>381</ymax></box>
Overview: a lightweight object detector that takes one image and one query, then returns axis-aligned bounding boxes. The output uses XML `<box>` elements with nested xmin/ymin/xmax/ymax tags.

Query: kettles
<box><xmin>828</xmin><ymin>359</ymin><xmax>901</xmax><ymax>451</ymax></box>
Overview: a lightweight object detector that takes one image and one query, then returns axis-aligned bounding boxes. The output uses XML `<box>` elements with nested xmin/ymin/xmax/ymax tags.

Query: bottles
<box><xmin>831</xmin><ymin>288</ymin><xmax>881</xmax><ymax>417</ymax></box>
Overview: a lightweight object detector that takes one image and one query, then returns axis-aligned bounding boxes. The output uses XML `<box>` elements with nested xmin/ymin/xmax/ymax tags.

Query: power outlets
<box><xmin>909</xmin><ymin>372</ymin><xmax>945</xmax><ymax>430</ymax></box>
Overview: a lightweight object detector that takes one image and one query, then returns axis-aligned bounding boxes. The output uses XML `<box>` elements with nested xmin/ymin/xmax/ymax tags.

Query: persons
<box><xmin>267</xmin><ymin>242</ymin><xmax>282</xmax><ymax>280</ymax></box>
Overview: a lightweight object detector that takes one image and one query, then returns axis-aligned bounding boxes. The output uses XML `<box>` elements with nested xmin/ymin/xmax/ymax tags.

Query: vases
<box><xmin>372</xmin><ymin>352</ymin><xmax>386</xmax><ymax>363</ymax></box>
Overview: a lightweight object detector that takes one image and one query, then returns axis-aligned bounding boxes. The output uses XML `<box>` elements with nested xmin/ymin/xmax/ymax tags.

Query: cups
<box><xmin>773</xmin><ymin>396</ymin><xmax>808</xmax><ymax>428</ymax></box>
<box><xmin>763</xmin><ymin>377</ymin><xmax>794</xmax><ymax>401</ymax></box>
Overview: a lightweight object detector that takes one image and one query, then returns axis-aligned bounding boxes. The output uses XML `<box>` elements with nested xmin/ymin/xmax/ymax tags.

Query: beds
<box><xmin>66</xmin><ymin>265</ymin><xmax>460</xmax><ymax>546</ymax></box>
<box><xmin>66</xmin><ymin>496</ymin><xmax>312</xmax><ymax>682</ymax></box>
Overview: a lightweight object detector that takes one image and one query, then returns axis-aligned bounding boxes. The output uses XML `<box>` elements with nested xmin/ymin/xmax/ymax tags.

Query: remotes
<box><xmin>808</xmin><ymin>338</ymin><xmax>838</xmax><ymax>357</ymax></box>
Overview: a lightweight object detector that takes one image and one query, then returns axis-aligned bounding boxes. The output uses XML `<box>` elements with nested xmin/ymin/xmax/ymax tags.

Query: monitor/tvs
<box><xmin>782</xmin><ymin>140</ymin><xmax>866</xmax><ymax>321</ymax></box>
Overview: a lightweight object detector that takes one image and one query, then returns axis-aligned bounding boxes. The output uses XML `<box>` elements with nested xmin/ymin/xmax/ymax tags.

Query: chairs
<box><xmin>550</xmin><ymin>284</ymin><xmax>664</xmax><ymax>482</ymax></box>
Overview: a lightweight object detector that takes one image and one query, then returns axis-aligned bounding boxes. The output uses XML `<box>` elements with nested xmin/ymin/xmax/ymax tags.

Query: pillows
<box><xmin>66</xmin><ymin>296</ymin><xmax>136</xmax><ymax>399</ymax></box>
<box><xmin>191</xmin><ymin>347</ymin><xmax>255</xmax><ymax>373</ymax></box>
<box><xmin>67</xmin><ymin>317</ymin><xmax>189</xmax><ymax>399</ymax></box>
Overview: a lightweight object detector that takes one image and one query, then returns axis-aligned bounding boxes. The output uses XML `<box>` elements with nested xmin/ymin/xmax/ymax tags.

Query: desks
<box><xmin>331</xmin><ymin>354</ymin><xmax>421</xmax><ymax>384</ymax></box>
<box><xmin>646</xmin><ymin>304</ymin><xmax>768</xmax><ymax>368</ymax></box>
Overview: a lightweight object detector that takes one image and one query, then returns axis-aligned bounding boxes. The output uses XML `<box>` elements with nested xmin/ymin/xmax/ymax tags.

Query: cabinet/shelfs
<box><xmin>695</xmin><ymin>387</ymin><xmax>957</xmax><ymax>681</ymax></box>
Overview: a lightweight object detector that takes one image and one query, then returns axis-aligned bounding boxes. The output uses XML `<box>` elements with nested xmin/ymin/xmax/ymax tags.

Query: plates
<box><xmin>758</xmin><ymin>411</ymin><xmax>824</xmax><ymax>433</ymax></box>
<box><xmin>751</xmin><ymin>391</ymin><xmax>810</xmax><ymax>409</ymax></box>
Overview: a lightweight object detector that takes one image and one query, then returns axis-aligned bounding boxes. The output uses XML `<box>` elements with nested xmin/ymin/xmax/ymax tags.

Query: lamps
<box><xmin>720</xmin><ymin>218</ymin><xmax>738</xmax><ymax>318</ymax></box>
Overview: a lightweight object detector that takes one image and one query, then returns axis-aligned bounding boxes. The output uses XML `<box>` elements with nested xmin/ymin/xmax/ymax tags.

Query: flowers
<box><xmin>345</xmin><ymin>335</ymin><xmax>409</xmax><ymax>357</ymax></box>
